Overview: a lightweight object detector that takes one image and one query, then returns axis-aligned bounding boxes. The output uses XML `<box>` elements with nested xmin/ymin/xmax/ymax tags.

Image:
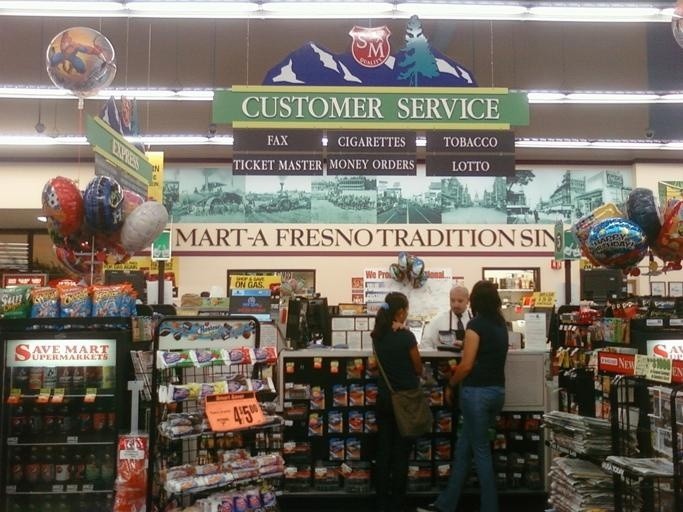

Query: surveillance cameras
<box><xmin>208</xmin><ymin>123</ymin><xmax>217</xmax><ymax>135</ymax></box>
<box><xmin>644</xmin><ymin>128</ymin><xmax>654</xmax><ymax>138</ymax></box>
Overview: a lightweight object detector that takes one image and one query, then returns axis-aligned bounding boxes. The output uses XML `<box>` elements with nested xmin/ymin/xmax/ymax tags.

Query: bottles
<box><xmin>9</xmin><ymin>406</ymin><xmax>115</xmax><ymax>512</ymax></box>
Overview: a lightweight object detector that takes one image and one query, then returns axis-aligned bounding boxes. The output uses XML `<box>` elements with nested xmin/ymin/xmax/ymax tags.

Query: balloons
<box><xmin>671</xmin><ymin>1</ymin><xmax>683</xmax><ymax>48</ymax></box>
<box><xmin>41</xmin><ymin>176</ymin><xmax>170</xmax><ymax>280</ymax></box>
<box><xmin>575</xmin><ymin>186</ymin><xmax>682</xmax><ymax>279</ymax></box>
<box><xmin>46</xmin><ymin>27</ymin><xmax>118</xmax><ymax>110</ymax></box>
<box><xmin>390</xmin><ymin>250</ymin><xmax>428</xmax><ymax>289</ymax></box>
<box><xmin>280</xmin><ymin>279</ymin><xmax>306</xmax><ymax>295</ymax></box>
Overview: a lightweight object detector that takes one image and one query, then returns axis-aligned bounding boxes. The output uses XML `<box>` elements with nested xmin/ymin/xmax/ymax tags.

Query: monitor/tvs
<box><xmin>286</xmin><ymin>297</ymin><xmax>330</xmax><ymax>347</ymax></box>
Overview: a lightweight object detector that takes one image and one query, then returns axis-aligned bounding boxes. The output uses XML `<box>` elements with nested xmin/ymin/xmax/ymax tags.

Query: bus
<box><xmin>507</xmin><ymin>205</ymin><xmax>530</xmax><ymax>218</ymax></box>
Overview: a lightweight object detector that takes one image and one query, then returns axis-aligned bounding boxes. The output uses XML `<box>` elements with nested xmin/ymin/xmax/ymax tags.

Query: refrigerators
<box><xmin>0</xmin><ymin>318</ymin><xmax>132</xmax><ymax>511</ymax></box>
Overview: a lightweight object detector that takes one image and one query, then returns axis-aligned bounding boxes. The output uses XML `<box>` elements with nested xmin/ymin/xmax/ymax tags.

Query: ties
<box><xmin>454</xmin><ymin>312</ymin><xmax>464</xmax><ymax>330</ymax></box>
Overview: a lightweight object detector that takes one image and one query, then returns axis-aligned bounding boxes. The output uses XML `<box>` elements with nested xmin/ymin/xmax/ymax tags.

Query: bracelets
<box><xmin>448</xmin><ymin>383</ymin><xmax>454</xmax><ymax>390</ymax></box>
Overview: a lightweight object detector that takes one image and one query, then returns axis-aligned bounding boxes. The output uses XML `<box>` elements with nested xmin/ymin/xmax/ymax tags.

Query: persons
<box><xmin>524</xmin><ymin>209</ymin><xmax>540</xmax><ymax>222</ymax></box>
<box><xmin>327</xmin><ymin>191</ymin><xmax>371</xmax><ymax>209</ymax></box>
<box><xmin>193</xmin><ymin>192</ymin><xmax>311</xmax><ymax>214</ymax></box>
<box><xmin>418</xmin><ymin>280</ymin><xmax>508</xmax><ymax>512</ymax></box>
<box><xmin>370</xmin><ymin>291</ymin><xmax>428</xmax><ymax>509</ymax></box>
<box><xmin>475</xmin><ymin>196</ymin><xmax>507</xmax><ymax>211</ymax></box>
<box><xmin>422</xmin><ymin>284</ymin><xmax>474</xmax><ymax>352</ymax></box>
<box><xmin>378</xmin><ymin>192</ymin><xmax>408</xmax><ymax>215</ymax></box>
<box><xmin>412</xmin><ymin>190</ymin><xmax>471</xmax><ymax>210</ymax></box>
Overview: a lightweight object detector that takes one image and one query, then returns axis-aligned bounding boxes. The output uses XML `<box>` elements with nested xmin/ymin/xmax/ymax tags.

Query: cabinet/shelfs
<box><xmin>0</xmin><ymin>305</ymin><xmax>182</xmax><ymax>511</ymax></box>
<box><xmin>551</xmin><ymin>296</ymin><xmax>682</xmax><ymax>512</ymax></box>
<box><xmin>276</xmin><ymin>351</ymin><xmax>548</xmax><ymax>499</ymax></box>
<box><xmin>150</xmin><ymin>311</ymin><xmax>287</xmax><ymax>512</ymax></box>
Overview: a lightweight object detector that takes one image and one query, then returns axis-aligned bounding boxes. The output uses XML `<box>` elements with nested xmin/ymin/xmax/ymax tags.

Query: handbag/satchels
<box><xmin>392</xmin><ymin>388</ymin><xmax>435</xmax><ymax>437</ymax></box>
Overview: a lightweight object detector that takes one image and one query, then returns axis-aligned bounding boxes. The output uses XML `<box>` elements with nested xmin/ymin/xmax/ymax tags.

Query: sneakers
<box><xmin>416</xmin><ymin>503</ymin><xmax>438</xmax><ymax>512</ymax></box>
